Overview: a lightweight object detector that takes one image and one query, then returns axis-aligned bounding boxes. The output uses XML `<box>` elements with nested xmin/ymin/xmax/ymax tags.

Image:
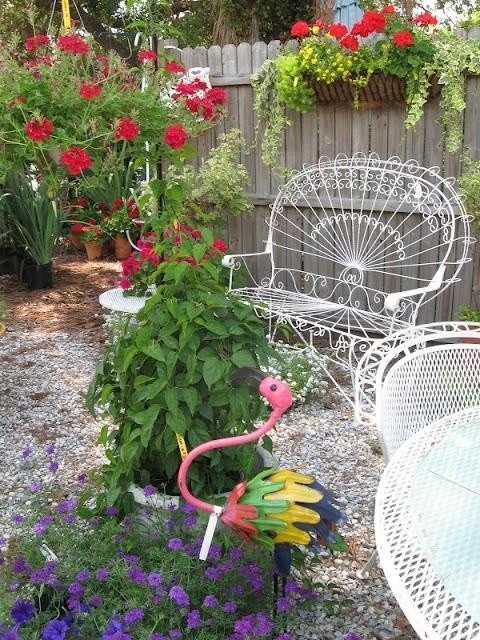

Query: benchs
<box><xmin>221</xmin><ymin>154</ymin><xmax>475</xmax><ymax>426</ymax></box>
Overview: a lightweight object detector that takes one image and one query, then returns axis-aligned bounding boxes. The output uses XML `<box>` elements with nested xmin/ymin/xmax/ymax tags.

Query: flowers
<box><xmin>19</xmin><ymin>28</ymin><xmax>228</xmax><ymax>174</ymax></box>
<box><xmin>288</xmin><ymin>7</ymin><xmax>440</xmax><ymax>84</ymax></box>
<box><xmin>67</xmin><ymin>196</ymin><xmax>229</xmax><ymax>293</ymax></box>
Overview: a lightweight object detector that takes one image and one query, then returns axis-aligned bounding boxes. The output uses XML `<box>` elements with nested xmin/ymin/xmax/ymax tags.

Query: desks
<box><xmin>98</xmin><ymin>282</ymin><xmax>157</xmax><ymax>339</ymax></box>
<box><xmin>374</xmin><ymin>399</ymin><xmax>478</xmax><ymax>640</ymax></box>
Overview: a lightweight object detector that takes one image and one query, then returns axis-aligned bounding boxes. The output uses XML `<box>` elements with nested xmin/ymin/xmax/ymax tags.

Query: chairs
<box><xmin>373</xmin><ymin>319</ymin><xmax>480</xmax><ymax>466</ymax></box>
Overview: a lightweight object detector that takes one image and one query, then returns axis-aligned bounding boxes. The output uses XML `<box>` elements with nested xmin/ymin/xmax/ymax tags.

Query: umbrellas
<box><xmin>331</xmin><ymin>0</ymin><xmax>389</xmax><ymax>51</ymax></box>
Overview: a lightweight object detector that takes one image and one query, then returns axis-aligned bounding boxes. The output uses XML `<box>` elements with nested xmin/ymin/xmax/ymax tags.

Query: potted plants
<box><xmin>86</xmin><ymin>226</ymin><xmax>283</xmax><ymax>547</ymax></box>
